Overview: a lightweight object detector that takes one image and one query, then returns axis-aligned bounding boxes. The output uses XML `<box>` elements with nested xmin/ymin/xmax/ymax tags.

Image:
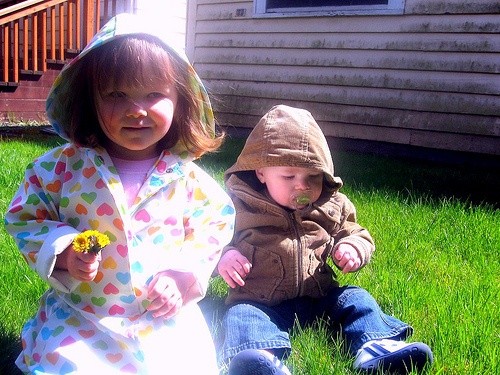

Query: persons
<box><xmin>214</xmin><ymin>104</ymin><xmax>433</xmax><ymax>375</ymax></box>
<box><xmin>4</xmin><ymin>12</ymin><xmax>236</xmax><ymax>375</ymax></box>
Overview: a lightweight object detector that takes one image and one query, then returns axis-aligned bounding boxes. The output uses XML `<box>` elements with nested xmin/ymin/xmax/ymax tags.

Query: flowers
<box><xmin>73</xmin><ymin>229</ymin><xmax>111</xmax><ymax>256</ymax></box>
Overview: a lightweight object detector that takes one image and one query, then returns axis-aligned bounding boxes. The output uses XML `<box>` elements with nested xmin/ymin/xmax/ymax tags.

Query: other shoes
<box><xmin>352</xmin><ymin>339</ymin><xmax>433</xmax><ymax>370</ymax></box>
<box><xmin>228</xmin><ymin>349</ymin><xmax>292</xmax><ymax>375</ymax></box>
